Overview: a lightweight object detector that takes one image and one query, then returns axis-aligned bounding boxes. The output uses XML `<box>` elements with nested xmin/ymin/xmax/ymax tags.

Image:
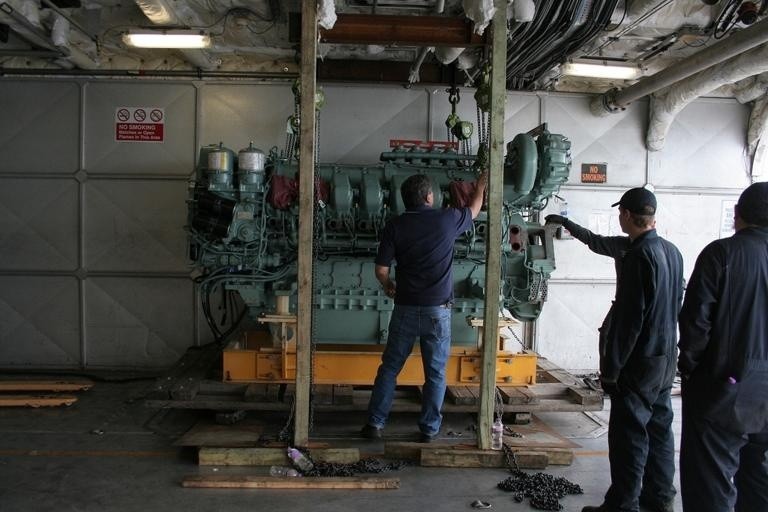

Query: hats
<box><xmin>611</xmin><ymin>187</ymin><xmax>658</xmax><ymax>215</ymax></box>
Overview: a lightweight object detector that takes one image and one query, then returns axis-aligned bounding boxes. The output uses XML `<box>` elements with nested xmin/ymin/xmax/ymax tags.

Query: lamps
<box><xmin>560</xmin><ymin>56</ymin><xmax>643</xmax><ymax>81</ymax></box>
<box><xmin>120</xmin><ymin>27</ymin><xmax>211</xmax><ymax>49</ymax></box>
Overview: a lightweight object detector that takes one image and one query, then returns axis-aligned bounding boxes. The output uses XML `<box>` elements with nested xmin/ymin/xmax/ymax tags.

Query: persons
<box><xmin>545</xmin><ymin>186</ymin><xmax>685</xmax><ymax>511</ymax></box>
<box><xmin>675</xmin><ymin>182</ymin><xmax>768</xmax><ymax>511</ymax></box>
<box><xmin>361</xmin><ymin>172</ymin><xmax>488</xmax><ymax>441</ymax></box>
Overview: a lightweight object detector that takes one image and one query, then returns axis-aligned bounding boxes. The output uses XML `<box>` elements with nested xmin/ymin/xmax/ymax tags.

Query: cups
<box><xmin>276</xmin><ymin>296</ymin><xmax>289</xmax><ymax>314</ymax></box>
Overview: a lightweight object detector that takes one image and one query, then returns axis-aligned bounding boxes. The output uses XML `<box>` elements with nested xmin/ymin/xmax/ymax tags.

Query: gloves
<box><xmin>544</xmin><ymin>213</ymin><xmax>568</xmax><ymax>226</ymax></box>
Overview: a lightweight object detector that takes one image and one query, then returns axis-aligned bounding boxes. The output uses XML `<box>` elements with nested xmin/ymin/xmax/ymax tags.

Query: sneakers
<box><xmin>639</xmin><ymin>495</ymin><xmax>674</xmax><ymax>512</ymax></box>
<box><xmin>580</xmin><ymin>500</ymin><xmax>630</xmax><ymax>512</ymax></box>
<box><xmin>421</xmin><ymin>428</ymin><xmax>441</xmax><ymax>444</ymax></box>
<box><xmin>361</xmin><ymin>425</ymin><xmax>384</xmax><ymax>440</ymax></box>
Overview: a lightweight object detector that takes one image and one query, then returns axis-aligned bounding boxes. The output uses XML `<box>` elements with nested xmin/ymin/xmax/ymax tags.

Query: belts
<box><xmin>439</xmin><ymin>303</ymin><xmax>451</xmax><ymax>308</ymax></box>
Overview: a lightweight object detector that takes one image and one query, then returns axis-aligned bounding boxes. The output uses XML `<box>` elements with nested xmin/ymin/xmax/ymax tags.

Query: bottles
<box><xmin>286</xmin><ymin>446</ymin><xmax>313</xmax><ymax>471</ymax></box>
<box><xmin>270</xmin><ymin>466</ymin><xmax>302</xmax><ymax>478</ymax></box>
<box><xmin>491</xmin><ymin>418</ymin><xmax>503</xmax><ymax>450</ymax></box>
